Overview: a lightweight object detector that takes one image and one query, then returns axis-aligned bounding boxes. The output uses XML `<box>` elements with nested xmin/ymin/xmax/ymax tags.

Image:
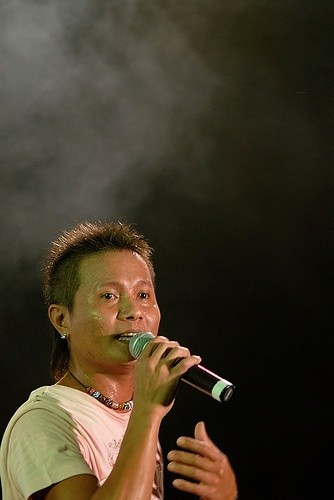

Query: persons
<box><xmin>0</xmin><ymin>222</ymin><xmax>238</xmax><ymax>500</ymax></box>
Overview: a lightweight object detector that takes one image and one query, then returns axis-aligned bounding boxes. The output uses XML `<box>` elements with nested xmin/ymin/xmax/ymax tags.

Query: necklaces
<box><xmin>67</xmin><ymin>368</ymin><xmax>134</xmax><ymax>412</ymax></box>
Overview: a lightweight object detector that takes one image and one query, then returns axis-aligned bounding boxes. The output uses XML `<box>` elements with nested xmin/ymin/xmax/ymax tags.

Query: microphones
<box><xmin>129</xmin><ymin>332</ymin><xmax>236</xmax><ymax>403</ymax></box>
